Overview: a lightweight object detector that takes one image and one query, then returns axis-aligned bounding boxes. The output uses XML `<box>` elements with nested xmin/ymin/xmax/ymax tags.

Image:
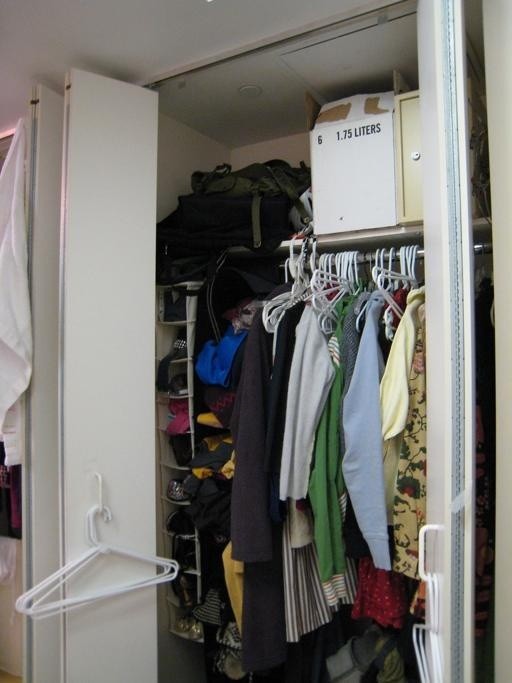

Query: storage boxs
<box><xmin>305</xmin><ymin>69</ymin><xmax>411</xmax><ymax>236</ymax></box>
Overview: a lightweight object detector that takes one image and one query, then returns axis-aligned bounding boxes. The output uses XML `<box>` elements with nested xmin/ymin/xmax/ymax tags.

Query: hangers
<box><xmin>14</xmin><ymin>503</ymin><xmax>182</xmax><ymax>622</ymax></box>
<box><xmin>261</xmin><ymin>244</ymin><xmax>414</xmax><ymax>338</ymax></box>
<box><xmin>413</xmin><ymin>572</ymin><xmax>448</xmax><ymax>683</ymax></box>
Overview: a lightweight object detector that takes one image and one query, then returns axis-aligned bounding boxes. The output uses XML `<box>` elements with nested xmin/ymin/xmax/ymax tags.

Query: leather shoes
<box><xmin>175</xmin><ymin>616</ymin><xmax>204</xmax><ymax>640</ymax></box>
<box><xmin>167</xmin><ymin>479</ymin><xmax>188</xmax><ymax>501</ymax></box>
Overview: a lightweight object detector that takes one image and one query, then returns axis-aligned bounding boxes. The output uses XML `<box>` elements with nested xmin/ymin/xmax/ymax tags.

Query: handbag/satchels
<box><xmin>191</xmin><ymin>159</ymin><xmax>310</xmax><ymax>198</ymax></box>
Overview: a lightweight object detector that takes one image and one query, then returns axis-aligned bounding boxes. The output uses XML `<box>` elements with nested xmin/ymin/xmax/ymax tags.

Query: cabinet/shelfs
<box><xmin>1</xmin><ymin>0</ymin><xmax>477</xmax><ymax>681</ymax></box>
